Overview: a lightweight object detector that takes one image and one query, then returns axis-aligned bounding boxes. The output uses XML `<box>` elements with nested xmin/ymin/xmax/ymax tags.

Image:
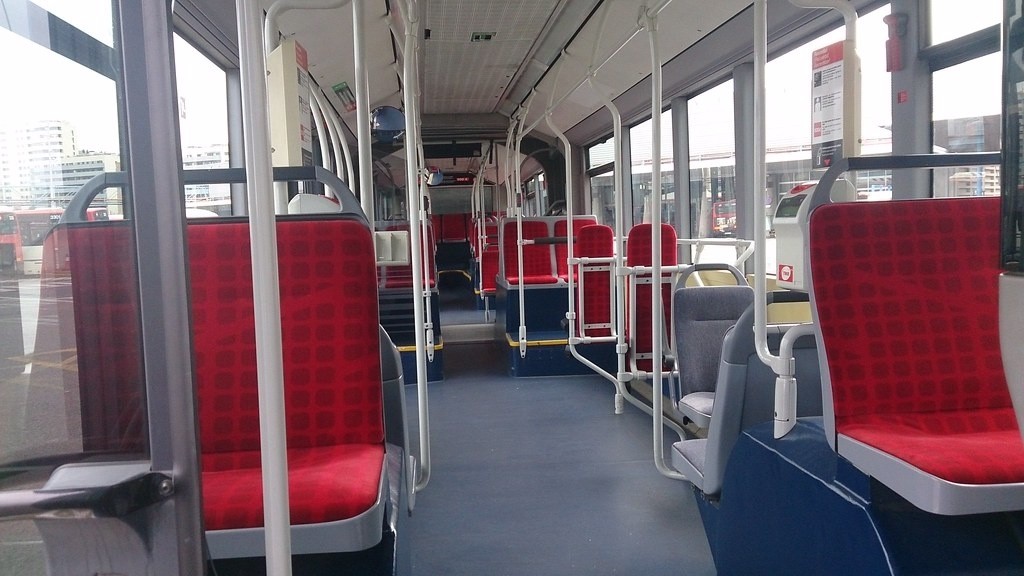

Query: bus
<box><xmin>0</xmin><ymin>208</ymin><xmax>109</xmax><ymax>277</ymax></box>
<box><xmin>522</xmin><ymin>141</ymin><xmax>949</xmax><ymax>278</ymax></box>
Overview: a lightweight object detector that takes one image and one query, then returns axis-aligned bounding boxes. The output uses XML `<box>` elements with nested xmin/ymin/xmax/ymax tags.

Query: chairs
<box><xmin>573</xmin><ymin>223</ymin><xmax>823</xmax><ymax>496</ymax></box>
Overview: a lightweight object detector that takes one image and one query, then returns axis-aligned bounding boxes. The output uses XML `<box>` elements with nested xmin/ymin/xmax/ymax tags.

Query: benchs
<box><xmin>430</xmin><ymin>211</ymin><xmax>598</xmax><ymax>291</ymax></box>
<box><xmin>375</xmin><ymin>217</ymin><xmax>437</xmax><ymax>292</ymax></box>
<box><xmin>18</xmin><ymin>164</ymin><xmax>389</xmax><ymax>560</ymax></box>
<box><xmin>797</xmin><ymin>150</ymin><xmax>1024</xmax><ymax>514</ymax></box>
